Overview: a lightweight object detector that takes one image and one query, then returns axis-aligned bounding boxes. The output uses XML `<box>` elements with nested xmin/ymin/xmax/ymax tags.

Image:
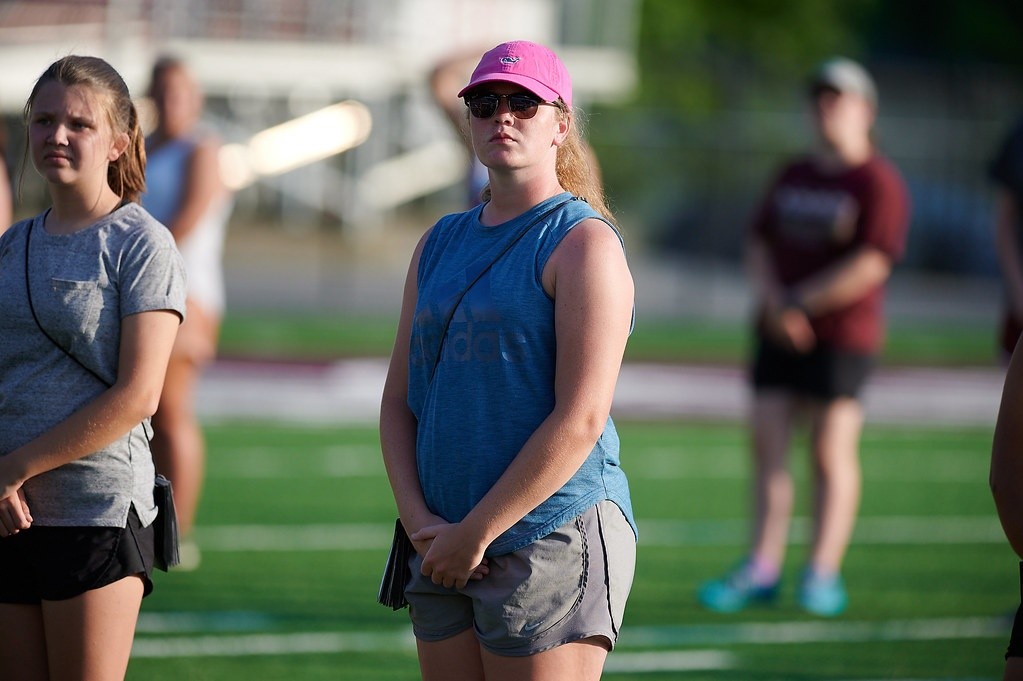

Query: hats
<box><xmin>807</xmin><ymin>55</ymin><xmax>878</xmax><ymax>102</ymax></box>
<box><xmin>458</xmin><ymin>40</ymin><xmax>572</xmax><ymax>112</ymax></box>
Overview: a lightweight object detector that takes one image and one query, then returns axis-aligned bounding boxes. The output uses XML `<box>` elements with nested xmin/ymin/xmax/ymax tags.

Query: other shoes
<box><xmin>795</xmin><ymin>568</ymin><xmax>849</xmax><ymax>617</ymax></box>
<box><xmin>698</xmin><ymin>561</ymin><xmax>783</xmax><ymax>615</ymax></box>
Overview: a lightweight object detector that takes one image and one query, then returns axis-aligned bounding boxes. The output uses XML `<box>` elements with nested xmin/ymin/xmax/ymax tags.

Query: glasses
<box><xmin>466</xmin><ymin>91</ymin><xmax>560</xmax><ymax>119</ymax></box>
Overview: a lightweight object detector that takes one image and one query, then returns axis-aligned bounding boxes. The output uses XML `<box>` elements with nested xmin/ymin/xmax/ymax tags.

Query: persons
<box><xmin>379</xmin><ymin>39</ymin><xmax>640</xmax><ymax>681</ymax></box>
<box><xmin>695</xmin><ymin>56</ymin><xmax>912</xmax><ymax>618</ymax></box>
<box><xmin>986</xmin><ymin>113</ymin><xmax>1023</xmax><ymax>363</ymax></box>
<box><xmin>988</xmin><ymin>331</ymin><xmax>1023</xmax><ymax>681</ymax></box>
<box><xmin>132</xmin><ymin>52</ymin><xmax>234</xmax><ymax>570</ymax></box>
<box><xmin>0</xmin><ymin>54</ymin><xmax>187</xmax><ymax>681</ymax></box>
